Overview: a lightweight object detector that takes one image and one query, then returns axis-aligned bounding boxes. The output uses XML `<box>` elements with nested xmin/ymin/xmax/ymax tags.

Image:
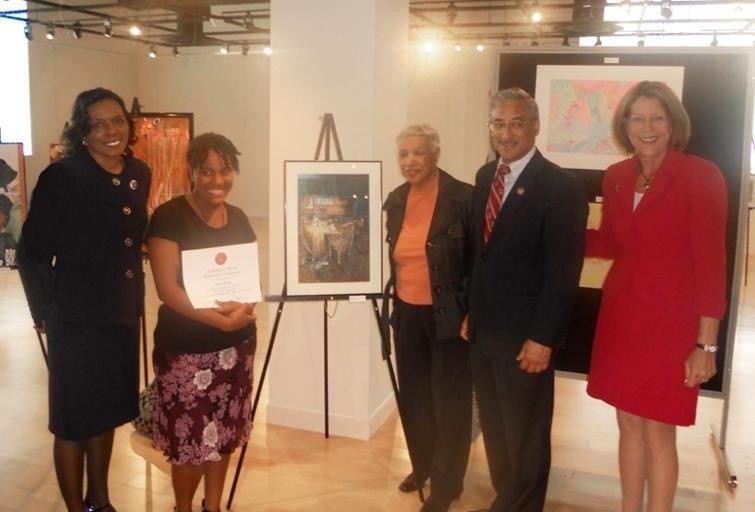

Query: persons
<box><xmin>380</xmin><ymin>123</ymin><xmax>475</xmax><ymax>512</ymax></box>
<box><xmin>582</xmin><ymin>81</ymin><xmax>728</xmax><ymax>512</ymax></box>
<box><xmin>15</xmin><ymin>86</ymin><xmax>153</xmax><ymax>512</ymax></box>
<box><xmin>454</xmin><ymin>88</ymin><xmax>590</xmax><ymax>512</ymax></box>
<box><xmin>147</xmin><ymin>134</ymin><xmax>258</xmax><ymax>512</ymax></box>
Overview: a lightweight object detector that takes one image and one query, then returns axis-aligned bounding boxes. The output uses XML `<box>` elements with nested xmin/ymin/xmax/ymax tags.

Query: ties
<box><xmin>484</xmin><ymin>164</ymin><xmax>511</xmax><ymax>245</ymax></box>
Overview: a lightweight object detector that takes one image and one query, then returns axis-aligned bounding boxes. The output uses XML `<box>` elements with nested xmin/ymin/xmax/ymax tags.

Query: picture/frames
<box><xmin>282</xmin><ymin>161</ymin><xmax>381</xmax><ymax>297</ymax></box>
<box><xmin>531</xmin><ymin>65</ymin><xmax>684</xmax><ymax>171</ymax></box>
<box><xmin>128</xmin><ymin>112</ymin><xmax>193</xmax><ymax>254</ymax></box>
<box><xmin>0</xmin><ymin>142</ymin><xmax>30</xmax><ymax>268</ymax></box>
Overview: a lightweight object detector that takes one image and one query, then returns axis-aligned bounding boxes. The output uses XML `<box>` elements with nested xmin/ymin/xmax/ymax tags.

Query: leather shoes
<box><xmin>399</xmin><ymin>473</ymin><xmax>453</xmax><ymax>511</ymax></box>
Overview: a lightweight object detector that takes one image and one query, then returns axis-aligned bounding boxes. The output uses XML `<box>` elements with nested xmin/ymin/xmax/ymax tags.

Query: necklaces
<box><xmin>191</xmin><ymin>195</ymin><xmax>225</xmax><ymax>229</ymax></box>
<box><xmin>640</xmin><ymin>166</ymin><xmax>652</xmax><ymax>191</ymax></box>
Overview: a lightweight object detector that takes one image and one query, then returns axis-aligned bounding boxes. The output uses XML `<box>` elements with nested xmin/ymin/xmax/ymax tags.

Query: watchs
<box><xmin>696</xmin><ymin>343</ymin><xmax>719</xmax><ymax>353</ymax></box>
